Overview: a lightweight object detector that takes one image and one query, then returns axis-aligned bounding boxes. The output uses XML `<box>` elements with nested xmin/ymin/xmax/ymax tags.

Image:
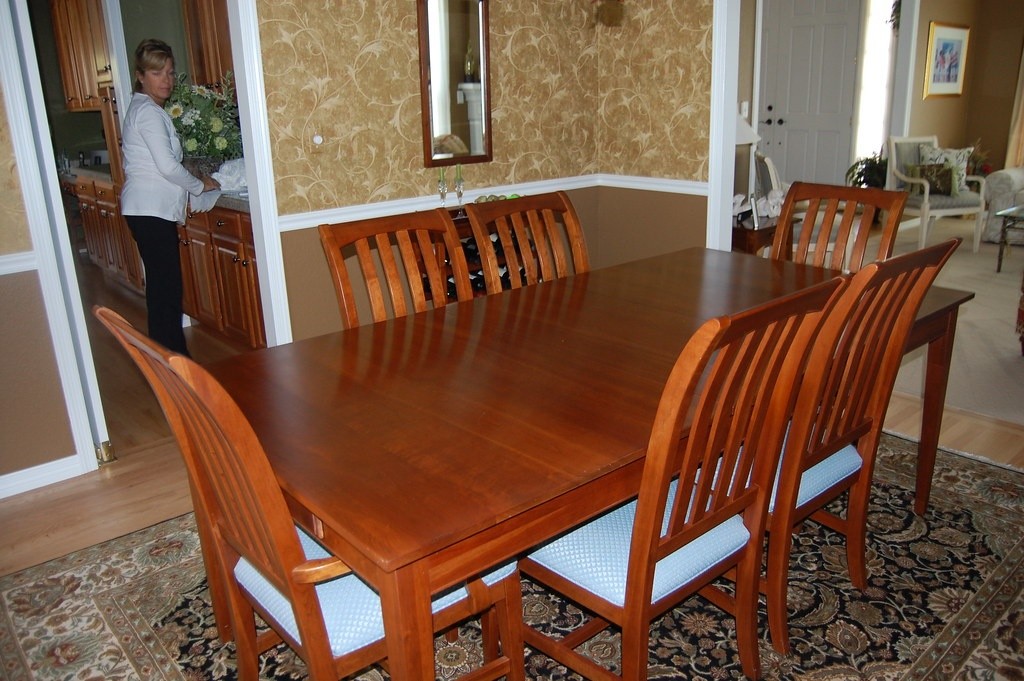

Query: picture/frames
<box><xmin>922</xmin><ymin>21</ymin><xmax>971</xmax><ymax>102</ymax></box>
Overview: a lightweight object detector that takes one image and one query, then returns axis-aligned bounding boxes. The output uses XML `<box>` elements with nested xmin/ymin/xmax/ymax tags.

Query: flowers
<box><xmin>162</xmin><ymin>70</ymin><xmax>245</xmax><ymax>163</ymax></box>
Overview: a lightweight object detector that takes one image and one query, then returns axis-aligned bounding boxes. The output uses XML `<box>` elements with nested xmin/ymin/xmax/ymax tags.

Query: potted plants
<box><xmin>844</xmin><ymin>144</ymin><xmax>889</xmax><ymax>225</ymax></box>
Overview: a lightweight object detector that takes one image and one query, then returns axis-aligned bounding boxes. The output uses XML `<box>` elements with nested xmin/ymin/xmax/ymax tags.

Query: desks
<box><xmin>995</xmin><ymin>204</ymin><xmax>1024</xmax><ymax>273</ymax></box>
<box><xmin>185</xmin><ymin>248</ymin><xmax>978</xmax><ymax>681</ymax></box>
<box><xmin>732</xmin><ymin>215</ymin><xmax>802</xmax><ymax>262</ymax></box>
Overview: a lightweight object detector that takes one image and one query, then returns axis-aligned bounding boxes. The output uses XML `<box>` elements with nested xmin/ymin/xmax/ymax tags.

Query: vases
<box><xmin>180</xmin><ymin>155</ymin><xmax>224</xmax><ymax>179</ymax></box>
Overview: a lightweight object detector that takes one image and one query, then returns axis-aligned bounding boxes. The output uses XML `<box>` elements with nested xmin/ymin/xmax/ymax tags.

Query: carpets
<box><xmin>0</xmin><ymin>427</ymin><xmax>1023</xmax><ymax>680</ymax></box>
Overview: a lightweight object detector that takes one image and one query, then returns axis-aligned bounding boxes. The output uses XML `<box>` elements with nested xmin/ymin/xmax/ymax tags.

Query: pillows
<box><xmin>905</xmin><ymin>144</ymin><xmax>975</xmax><ymax>200</ymax></box>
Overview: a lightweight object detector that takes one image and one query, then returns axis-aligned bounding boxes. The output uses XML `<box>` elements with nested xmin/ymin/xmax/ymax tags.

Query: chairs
<box><xmin>318</xmin><ymin>207</ymin><xmax>475</xmax><ymax>330</ymax></box>
<box><xmin>90</xmin><ymin>302</ymin><xmax>524</xmax><ymax>681</ymax></box>
<box><xmin>464</xmin><ymin>190</ymin><xmax>590</xmax><ymax>299</ymax></box>
<box><xmin>480</xmin><ymin>237</ymin><xmax>962</xmax><ymax>681</ymax></box>
<box><xmin>890</xmin><ymin>135</ymin><xmax>987</xmax><ymax>249</ymax></box>
<box><xmin>771</xmin><ymin>179</ymin><xmax>908</xmax><ymax>275</ymax></box>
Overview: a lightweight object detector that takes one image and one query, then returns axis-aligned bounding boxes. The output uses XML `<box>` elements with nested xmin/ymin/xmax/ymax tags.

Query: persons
<box><xmin>119</xmin><ymin>37</ymin><xmax>220</xmax><ymax>361</ymax></box>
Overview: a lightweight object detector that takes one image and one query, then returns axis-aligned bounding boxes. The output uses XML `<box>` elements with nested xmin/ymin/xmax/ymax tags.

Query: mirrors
<box><xmin>416</xmin><ymin>0</ymin><xmax>493</xmax><ymax>169</ymax></box>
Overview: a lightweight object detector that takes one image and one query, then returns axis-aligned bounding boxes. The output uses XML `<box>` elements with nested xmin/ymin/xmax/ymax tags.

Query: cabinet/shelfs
<box><xmin>49</xmin><ymin>0</ymin><xmax>147</xmax><ymax>299</ymax></box>
<box><xmin>176</xmin><ymin>207</ymin><xmax>267</xmax><ymax>349</ymax></box>
<box><xmin>183</xmin><ymin>0</ymin><xmax>237</xmax><ymax>107</ymax></box>
<box><xmin>408</xmin><ymin>209</ymin><xmax>537</xmax><ymax>305</ymax></box>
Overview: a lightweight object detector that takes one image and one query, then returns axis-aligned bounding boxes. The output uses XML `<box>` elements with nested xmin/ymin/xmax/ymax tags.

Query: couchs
<box><xmin>981</xmin><ymin>166</ymin><xmax>1024</xmax><ymax>243</ymax></box>
